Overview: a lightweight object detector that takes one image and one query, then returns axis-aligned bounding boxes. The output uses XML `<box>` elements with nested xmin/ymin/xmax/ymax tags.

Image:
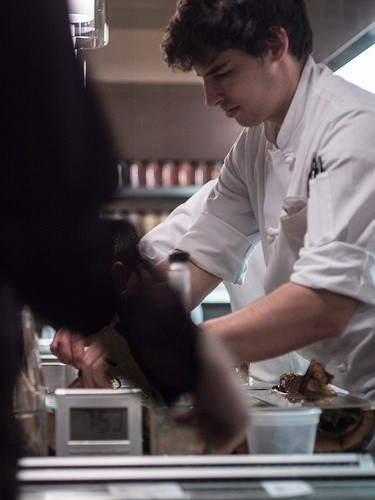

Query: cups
<box><xmin>245</xmin><ymin>406</ymin><xmax>322</xmax><ymax>454</ymax></box>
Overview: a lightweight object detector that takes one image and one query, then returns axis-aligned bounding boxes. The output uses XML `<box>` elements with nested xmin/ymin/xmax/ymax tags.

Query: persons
<box><xmin>0</xmin><ymin>1</ymin><xmax>247</xmax><ymax>500</ymax></box>
<box><xmin>49</xmin><ymin>2</ymin><xmax>375</xmax><ymax>441</ymax></box>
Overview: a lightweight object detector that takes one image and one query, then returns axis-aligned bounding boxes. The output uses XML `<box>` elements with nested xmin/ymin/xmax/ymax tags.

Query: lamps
<box><xmin>64</xmin><ymin>0</ymin><xmax>108</xmax><ymax>50</ymax></box>
